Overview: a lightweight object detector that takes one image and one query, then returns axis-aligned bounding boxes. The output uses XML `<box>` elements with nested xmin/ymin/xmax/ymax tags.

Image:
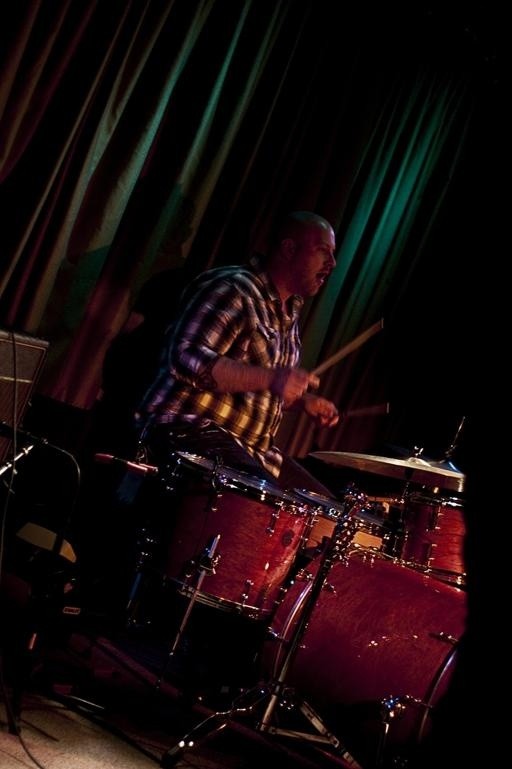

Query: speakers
<box><xmin>0</xmin><ymin>331</ymin><xmax>50</xmax><ymax>467</ymax></box>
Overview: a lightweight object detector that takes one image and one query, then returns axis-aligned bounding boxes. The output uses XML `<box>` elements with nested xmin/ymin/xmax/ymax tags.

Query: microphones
<box><xmin>1</xmin><ymin>417</ymin><xmax>49</xmax><ymax>454</ymax></box>
<box><xmin>0</xmin><ymin>443</ymin><xmax>36</xmax><ymax>477</ymax></box>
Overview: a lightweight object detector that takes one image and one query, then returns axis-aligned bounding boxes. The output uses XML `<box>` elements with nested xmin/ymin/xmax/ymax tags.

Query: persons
<box><xmin>78</xmin><ymin>215</ymin><xmax>341</xmax><ymax>635</ymax></box>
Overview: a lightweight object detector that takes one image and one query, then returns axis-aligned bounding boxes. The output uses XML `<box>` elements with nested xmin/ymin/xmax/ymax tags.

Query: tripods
<box><xmin>159</xmin><ymin>514</ymin><xmax>370</xmax><ymax>768</ymax></box>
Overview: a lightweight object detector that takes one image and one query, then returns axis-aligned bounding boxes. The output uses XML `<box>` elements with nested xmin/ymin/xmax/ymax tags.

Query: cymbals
<box><xmin>314</xmin><ymin>452</ymin><xmax>464</xmax><ymax>479</ymax></box>
<box><xmin>309</xmin><ymin>452</ymin><xmax>466</xmax><ymax>492</ymax></box>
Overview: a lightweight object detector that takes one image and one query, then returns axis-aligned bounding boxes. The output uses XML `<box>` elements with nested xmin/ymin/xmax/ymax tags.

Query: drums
<box><xmin>291</xmin><ymin>488</ymin><xmax>405</xmax><ymax>559</ymax></box>
<box><xmin>126</xmin><ymin>450</ymin><xmax>316</xmax><ymax>621</ymax></box>
<box><xmin>400</xmin><ymin>493</ymin><xmax>470</xmax><ymax>588</ymax></box>
<box><xmin>262</xmin><ymin>543</ymin><xmax>469</xmax><ymax>765</ymax></box>
